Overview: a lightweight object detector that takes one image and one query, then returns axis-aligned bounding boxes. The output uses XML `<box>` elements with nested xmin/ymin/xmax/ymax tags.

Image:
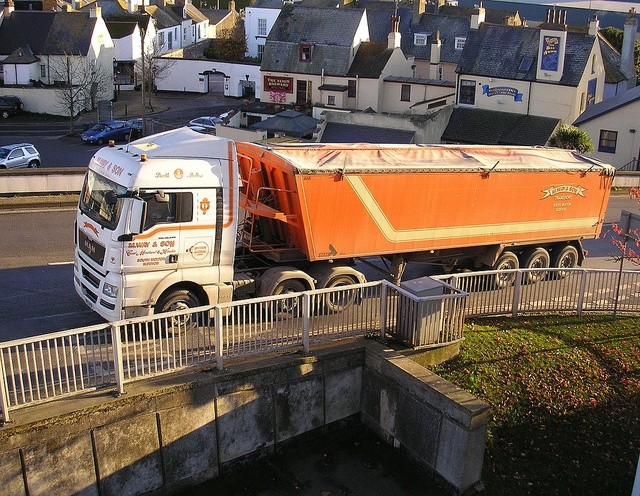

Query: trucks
<box><xmin>73</xmin><ymin>125</ymin><xmax>617</xmax><ymax>336</ymax></box>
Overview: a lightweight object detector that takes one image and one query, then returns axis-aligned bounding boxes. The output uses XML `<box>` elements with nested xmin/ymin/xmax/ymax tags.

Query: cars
<box><xmin>219</xmin><ymin>109</ymin><xmax>234</xmax><ymax>120</ymax></box>
<box><xmin>189</xmin><ymin>116</ymin><xmax>223</xmax><ymax>128</ymax></box>
<box><xmin>79</xmin><ymin>121</ymin><xmax>142</xmax><ymax>145</ymax></box>
<box><xmin>127</xmin><ymin>118</ymin><xmax>142</xmax><ymax>127</ymax></box>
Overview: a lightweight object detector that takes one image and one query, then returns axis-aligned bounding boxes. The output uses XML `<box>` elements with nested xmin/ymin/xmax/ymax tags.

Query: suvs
<box><xmin>0</xmin><ymin>143</ymin><xmax>41</xmax><ymax>169</ymax></box>
